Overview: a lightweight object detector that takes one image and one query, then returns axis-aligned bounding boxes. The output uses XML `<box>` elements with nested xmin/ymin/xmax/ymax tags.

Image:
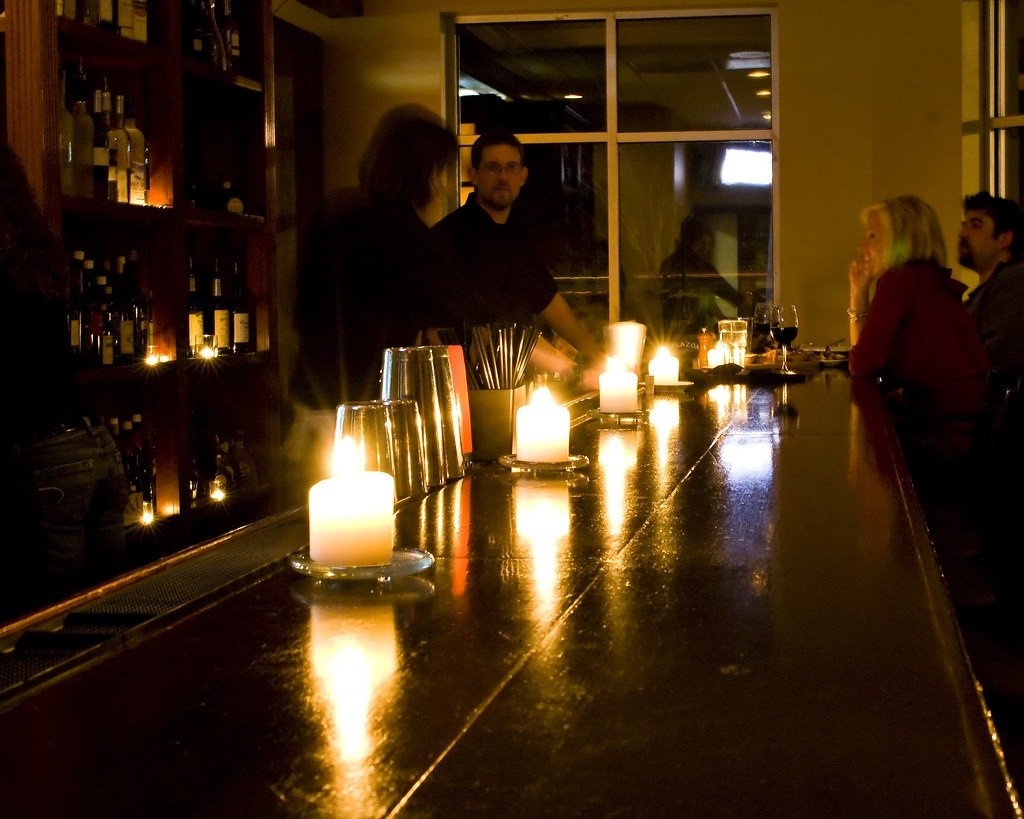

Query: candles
<box><xmin>599</xmin><ymin>356</ymin><xmax>640</xmax><ymax>415</ymax></box>
<box><xmin>649</xmin><ymin>346</ymin><xmax>679</xmax><ymax>385</ymax></box>
<box><xmin>707</xmin><ymin>342</ymin><xmax>725</xmax><ymax>368</ymax></box>
<box><xmin>307</xmin><ymin>435</ymin><xmax>396</xmax><ymax>569</ymax></box>
<box><xmin>514</xmin><ymin>385</ymin><xmax>570</xmax><ymax>463</ymax></box>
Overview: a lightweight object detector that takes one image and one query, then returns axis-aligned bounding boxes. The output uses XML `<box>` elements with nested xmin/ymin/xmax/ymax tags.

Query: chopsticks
<box><xmin>463</xmin><ymin>321</ymin><xmax>543</xmax><ymax>390</ymax></box>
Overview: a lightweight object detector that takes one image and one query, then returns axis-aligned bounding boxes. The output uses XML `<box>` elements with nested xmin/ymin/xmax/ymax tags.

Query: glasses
<box><xmin>479</xmin><ymin>160</ymin><xmax>522</xmax><ymax>176</ymax></box>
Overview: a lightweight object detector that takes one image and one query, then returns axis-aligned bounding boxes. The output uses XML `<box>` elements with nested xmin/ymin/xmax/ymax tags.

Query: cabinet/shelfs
<box><xmin>0</xmin><ymin>0</ymin><xmax>285</xmax><ymax>633</ymax></box>
<box><xmin>477</xmin><ymin>98</ymin><xmax>595</xmax><ymax>279</ymax></box>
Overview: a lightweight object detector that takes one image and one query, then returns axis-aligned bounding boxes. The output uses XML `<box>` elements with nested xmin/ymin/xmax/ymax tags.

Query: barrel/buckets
<box><xmin>22</xmin><ymin>413</ymin><xmax>130</xmax><ymax>534</ymax></box>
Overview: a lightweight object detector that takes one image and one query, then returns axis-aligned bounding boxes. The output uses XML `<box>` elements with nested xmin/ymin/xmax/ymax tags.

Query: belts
<box><xmin>5</xmin><ymin>419</ymin><xmax>102</xmax><ymax>451</ymax></box>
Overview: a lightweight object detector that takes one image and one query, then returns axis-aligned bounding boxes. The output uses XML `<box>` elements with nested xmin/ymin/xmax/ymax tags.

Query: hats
<box><xmin>680</xmin><ymin>215</ymin><xmax>714</xmax><ymax>243</ymax></box>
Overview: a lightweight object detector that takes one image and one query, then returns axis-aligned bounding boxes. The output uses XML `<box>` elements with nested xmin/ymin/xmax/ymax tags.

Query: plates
<box><xmin>817</xmin><ymin>359</ymin><xmax>847</xmax><ymax>367</ymax></box>
<box><xmin>638</xmin><ymin>381</ymin><xmax>695</xmax><ymax>393</ymax></box>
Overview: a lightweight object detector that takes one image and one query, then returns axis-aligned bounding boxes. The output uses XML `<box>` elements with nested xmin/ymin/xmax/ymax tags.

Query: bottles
<box><xmin>187</xmin><ymin>255</ymin><xmax>252</xmax><ymax>357</ymax></box>
<box><xmin>55</xmin><ymin>0</ymin><xmax>151</xmax><ymax>43</ymax></box>
<box><xmin>68</xmin><ymin>247</ymin><xmax>155</xmax><ymax>367</ymax></box>
<box><xmin>92</xmin><ymin>412</ymin><xmax>158</xmax><ymax>525</ymax></box>
<box><xmin>695</xmin><ymin>325</ymin><xmax>713</xmax><ymax>368</ymax></box>
<box><xmin>181</xmin><ymin>0</ymin><xmax>242</xmax><ymax>72</ymax></box>
<box><xmin>188</xmin><ymin>425</ymin><xmax>258</xmax><ymax>505</ymax></box>
<box><xmin>59</xmin><ymin>58</ymin><xmax>147</xmax><ymax>205</ymax></box>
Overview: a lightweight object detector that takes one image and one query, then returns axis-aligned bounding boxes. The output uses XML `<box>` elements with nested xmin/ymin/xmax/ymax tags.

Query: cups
<box><xmin>717</xmin><ymin>316</ymin><xmax>753</xmax><ymax>368</ymax></box>
<box><xmin>332</xmin><ymin>344</ymin><xmax>533</xmax><ymax>497</ymax></box>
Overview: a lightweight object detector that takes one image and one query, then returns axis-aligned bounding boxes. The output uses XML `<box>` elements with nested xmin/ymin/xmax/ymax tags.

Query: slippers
<box><xmin>955</xmin><ymin>217</ymin><xmax>998</xmax><ymax>237</ymax></box>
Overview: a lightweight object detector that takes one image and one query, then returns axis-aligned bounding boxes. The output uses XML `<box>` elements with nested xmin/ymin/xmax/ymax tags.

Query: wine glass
<box><xmin>751</xmin><ymin>302</ymin><xmax>772</xmax><ymax>354</ymax></box>
<box><xmin>771</xmin><ymin>304</ymin><xmax>800</xmax><ymax>375</ymax></box>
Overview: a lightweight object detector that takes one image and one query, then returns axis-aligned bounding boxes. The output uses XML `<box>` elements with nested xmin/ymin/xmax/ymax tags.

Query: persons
<box><xmin>955</xmin><ymin>189</ymin><xmax>1024</xmax><ymax>655</ymax></box>
<box><xmin>848</xmin><ymin>198</ymin><xmax>1024</xmax><ymax>482</ymax></box>
<box><xmin>660</xmin><ymin>214</ymin><xmax>752</xmax><ymax>336</ymax></box>
<box><xmin>0</xmin><ymin>140</ymin><xmax>129</xmax><ymax>600</ymax></box>
<box><xmin>286</xmin><ymin>100</ymin><xmax>493</xmax><ymax>435</ymax></box>
<box><xmin>424</xmin><ymin>132</ymin><xmax>600</xmax><ymax>375</ymax></box>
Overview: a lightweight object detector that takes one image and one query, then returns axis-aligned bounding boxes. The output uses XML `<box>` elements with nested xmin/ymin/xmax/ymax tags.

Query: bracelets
<box><xmin>847</xmin><ymin>308</ymin><xmax>871</xmax><ymax>321</ymax></box>
